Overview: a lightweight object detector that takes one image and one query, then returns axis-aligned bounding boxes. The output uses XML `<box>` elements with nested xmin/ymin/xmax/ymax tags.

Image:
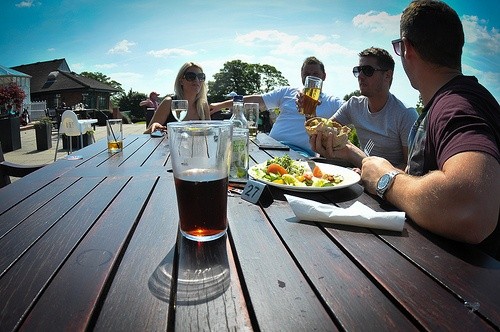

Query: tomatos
<box><xmin>313</xmin><ymin>166</ymin><xmax>322</xmax><ymax>176</ymax></box>
<box><xmin>266</xmin><ymin>164</ymin><xmax>287</xmax><ymax>175</ymax></box>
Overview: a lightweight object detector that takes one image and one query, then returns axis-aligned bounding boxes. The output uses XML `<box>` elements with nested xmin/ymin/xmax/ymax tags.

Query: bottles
<box><xmin>229</xmin><ymin>94</ymin><xmax>248</xmax><ymax>128</ymax></box>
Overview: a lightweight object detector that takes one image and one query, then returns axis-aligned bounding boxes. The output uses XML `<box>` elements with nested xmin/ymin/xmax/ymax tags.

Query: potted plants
<box><xmin>0</xmin><ymin>82</ymin><xmax>26</xmax><ymax>153</ymax></box>
<box><xmin>63</xmin><ymin>130</ymin><xmax>95</xmax><ymax>151</ymax></box>
<box><xmin>33</xmin><ymin>115</ymin><xmax>54</xmax><ymax>153</ymax></box>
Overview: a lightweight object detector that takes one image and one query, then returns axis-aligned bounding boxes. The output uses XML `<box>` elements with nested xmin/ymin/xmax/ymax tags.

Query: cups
<box><xmin>244</xmin><ymin>102</ymin><xmax>260</xmax><ymax>140</ymax></box>
<box><xmin>167</xmin><ymin>121</ymin><xmax>233</xmax><ymax>242</ymax></box>
<box><xmin>106</xmin><ymin>119</ymin><xmax>123</xmax><ymax>154</ymax></box>
<box><xmin>298</xmin><ymin>76</ymin><xmax>323</xmax><ymax>117</ymax></box>
<box><xmin>231</xmin><ymin>127</ymin><xmax>250</xmax><ymax>179</ymax></box>
<box><xmin>171</xmin><ymin>100</ymin><xmax>188</xmax><ymax>122</ymax></box>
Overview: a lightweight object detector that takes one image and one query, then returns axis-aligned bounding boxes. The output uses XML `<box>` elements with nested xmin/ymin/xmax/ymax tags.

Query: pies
<box><xmin>303</xmin><ymin>117</ymin><xmax>352</xmax><ymax>151</ymax></box>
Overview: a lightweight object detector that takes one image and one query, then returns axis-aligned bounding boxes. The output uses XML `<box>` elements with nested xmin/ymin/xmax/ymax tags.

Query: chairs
<box><xmin>53</xmin><ymin>110</ymin><xmax>98</xmax><ymax>162</ymax></box>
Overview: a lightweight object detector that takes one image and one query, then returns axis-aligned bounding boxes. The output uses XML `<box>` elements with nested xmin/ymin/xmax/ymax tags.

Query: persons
<box><xmin>215</xmin><ymin>56</ymin><xmax>347</xmax><ymax>161</ymax></box>
<box><xmin>308</xmin><ymin>0</ymin><xmax>500</xmax><ymax>263</ymax></box>
<box><xmin>329</xmin><ymin>47</ymin><xmax>420</xmax><ymax>168</ymax></box>
<box><xmin>143</xmin><ymin>61</ymin><xmax>211</xmax><ymax>133</ymax></box>
<box><xmin>140</xmin><ymin>91</ymin><xmax>160</xmax><ymax>110</ymax></box>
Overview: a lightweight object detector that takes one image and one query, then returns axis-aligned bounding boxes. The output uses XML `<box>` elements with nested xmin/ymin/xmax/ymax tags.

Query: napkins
<box><xmin>285</xmin><ymin>195</ymin><xmax>405</xmax><ymax>234</ymax></box>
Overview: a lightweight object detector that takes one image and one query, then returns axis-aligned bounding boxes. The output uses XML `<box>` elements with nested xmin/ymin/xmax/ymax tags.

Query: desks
<box><xmin>0</xmin><ymin>133</ymin><xmax>500</xmax><ymax>332</ymax></box>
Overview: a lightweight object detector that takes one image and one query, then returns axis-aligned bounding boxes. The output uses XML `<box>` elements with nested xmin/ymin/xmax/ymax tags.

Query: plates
<box><xmin>247</xmin><ymin>161</ymin><xmax>362</xmax><ymax>192</ymax></box>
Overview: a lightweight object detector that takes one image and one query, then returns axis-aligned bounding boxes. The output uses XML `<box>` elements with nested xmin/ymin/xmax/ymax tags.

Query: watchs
<box><xmin>376</xmin><ymin>169</ymin><xmax>405</xmax><ymax>204</ymax></box>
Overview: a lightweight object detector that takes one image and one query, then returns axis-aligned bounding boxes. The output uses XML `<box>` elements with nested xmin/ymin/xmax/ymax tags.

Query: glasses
<box><xmin>181</xmin><ymin>71</ymin><xmax>205</xmax><ymax>82</ymax></box>
<box><xmin>392</xmin><ymin>39</ymin><xmax>405</xmax><ymax>56</ymax></box>
<box><xmin>353</xmin><ymin>65</ymin><xmax>387</xmax><ymax>78</ymax></box>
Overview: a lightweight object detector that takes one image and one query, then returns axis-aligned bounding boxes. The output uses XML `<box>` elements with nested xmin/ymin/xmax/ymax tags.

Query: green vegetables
<box><xmin>255</xmin><ymin>155</ymin><xmax>333</xmax><ymax>187</ymax></box>
